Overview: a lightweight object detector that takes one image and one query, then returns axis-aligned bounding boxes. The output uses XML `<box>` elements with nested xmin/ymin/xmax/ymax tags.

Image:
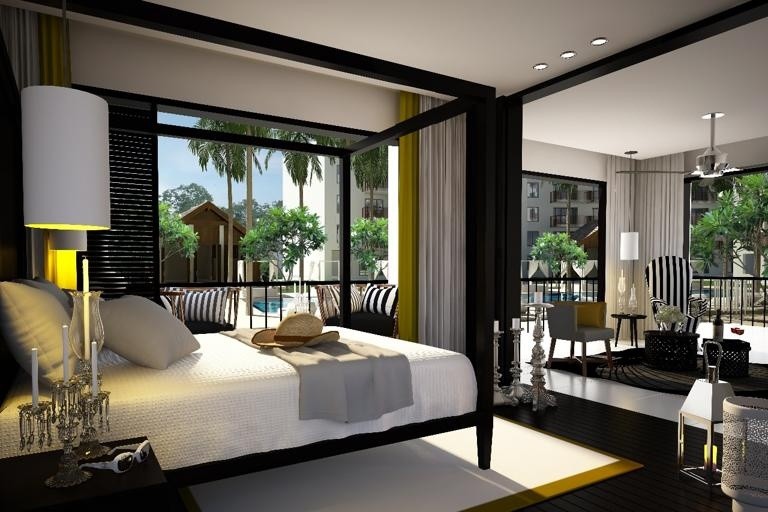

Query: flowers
<box><xmin>654</xmin><ymin>306</ymin><xmax>683</xmax><ymax>322</ymax></box>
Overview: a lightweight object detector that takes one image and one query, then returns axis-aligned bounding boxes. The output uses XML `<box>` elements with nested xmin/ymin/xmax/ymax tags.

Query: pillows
<box><xmin>183</xmin><ymin>289</ymin><xmax>226</xmax><ymax>325</ymax></box>
<box><xmin>553</xmin><ymin>299</ymin><xmax>605</xmax><ymax>329</ymax></box>
<box><xmin>362</xmin><ymin>281</ymin><xmax>397</xmax><ymax>318</ymax></box>
<box><xmin>327</xmin><ymin>285</ymin><xmax>364</xmax><ymax>317</ymax></box>
<box><xmin>93</xmin><ymin>292</ymin><xmax>202</xmax><ymax>368</ymax></box>
<box><xmin>2</xmin><ymin>281</ymin><xmax>80</xmax><ymax>382</ymax></box>
<box><xmin>21</xmin><ymin>278</ymin><xmax>74</xmax><ymax>326</ymax></box>
<box><xmin>166</xmin><ymin>295</ymin><xmax>184</xmax><ymax>322</ymax></box>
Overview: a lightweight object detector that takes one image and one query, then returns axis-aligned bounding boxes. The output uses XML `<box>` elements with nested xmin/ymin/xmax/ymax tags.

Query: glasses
<box><xmin>82</xmin><ymin>440</ymin><xmax>151</xmax><ymax>473</ymax></box>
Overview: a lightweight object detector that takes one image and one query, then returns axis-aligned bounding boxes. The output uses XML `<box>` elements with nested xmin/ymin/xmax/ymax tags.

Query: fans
<box><xmin>613</xmin><ymin>114</ymin><xmax>768</xmax><ymax>188</ymax></box>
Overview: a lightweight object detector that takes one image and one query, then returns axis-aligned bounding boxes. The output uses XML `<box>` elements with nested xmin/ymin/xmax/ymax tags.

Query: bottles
<box><xmin>284</xmin><ymin>293</ymin><xmax>309</xmax><ymax>318</ymax></box>
<box><xmin>713</xmin><ymin>308</ymin><xmax>724</xmax><ymax>342</ymax></box>
<box><xmin>628</xmin><ymin>288</ymin><xmax>638</xmax><ymax>315</ymax></box>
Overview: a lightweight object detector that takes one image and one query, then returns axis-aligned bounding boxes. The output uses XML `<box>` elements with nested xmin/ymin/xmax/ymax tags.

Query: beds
<box><xmin>3</xmin><ymin>321</ymin><xmax>492</xmax><ymax>487</ymax></box>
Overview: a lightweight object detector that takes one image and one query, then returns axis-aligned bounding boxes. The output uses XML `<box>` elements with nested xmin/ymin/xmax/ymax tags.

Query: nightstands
<box><xmin>0</xmin><ymin>435</ymin><xmax>192</xmax><ymax>512</ymax></box>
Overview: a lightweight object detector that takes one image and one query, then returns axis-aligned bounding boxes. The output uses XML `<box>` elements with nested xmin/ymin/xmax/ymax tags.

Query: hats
<box><xmin>252</xmin><ymin>309</ymin><xmax>340</xmax><ymax>349</ymax></box>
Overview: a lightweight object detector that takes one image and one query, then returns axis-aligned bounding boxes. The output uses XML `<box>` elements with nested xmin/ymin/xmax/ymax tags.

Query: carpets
<box><xmin>188</xmin><ymin>412</ymin><xmax>644</xmax><ymax>511</ymax></box>
<box><xmin>525</xmin><ymin>349</ymin><xmax>768</xmax><ymax>398</ymax></box>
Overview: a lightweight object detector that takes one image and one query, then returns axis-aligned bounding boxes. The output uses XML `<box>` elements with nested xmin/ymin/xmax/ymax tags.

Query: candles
<box><xmin>304</xmin><ymin>282</ymin><xmax>306</xmax><ymax>294</ymax></box>
<box><xmin>61</xmin><ymin>320</ymin><xmax>70</xmax><ymax>387</ymax></box>
<box><xmin>293</xmin><ymin>282</ymin><xmax>296</xmax><ymax>295</ymax></box>
<box><xmin>493</xmin><ymin>320</ymin><xmax>500</xmax><ymax>333</ymax></box>
<box><xmin>82</xmin><ymin>254</ymin><xmax>90</xmax><ymax>359</ymax></box>
<box><xmin>299</xmin><ymin>278</ymin><xmax>302</xmax><ymax>294</ymax></box>
<box><xmin>534</xmin><ymin>291</ymin><xmax>543</xmax><ymax>303</ymax></box>
<box><xmin>31</xmin><ymin>347</ymin><xmax>39</xmax><ymax>408</ymax></box>
<box><xmin>91</xmin><ymin>337</ymin><xmax>98</xmax><ymax>397</ymax></box>
<box><xmin>512</xmin><ymin>317</ymin><xmax>520</xmax><ymax>330</ymax></box>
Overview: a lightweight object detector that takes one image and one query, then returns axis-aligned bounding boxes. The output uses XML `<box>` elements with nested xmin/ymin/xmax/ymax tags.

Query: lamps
<box><xmin>20</xmin><ymin>86</ymin><xmax>115</xmax><ymax>232</ymax></box>
<box><xmin>50</xmin><ymin>228</ymin><xmax>88</xmax><ymax>252</ymax></box>
<box><xmin>620</xmin><ymin>149</ymin><xmax>638</xmax><ymax>261</ymax></box>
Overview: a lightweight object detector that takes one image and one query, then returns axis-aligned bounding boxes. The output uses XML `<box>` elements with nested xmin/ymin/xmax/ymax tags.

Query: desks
<box><xmin>643</xmin><ymin>329</ymin><xmax>700</xmax><ymax>371</ymax></box>
<box><xmin>701</xmin><ymin>338</ymin><xmax>752</xmax><ymax>377</ymax></box>
<box><xmin>610</xmin><ymin>312</ymin><xmax>648</xmax><ymax>348</ymax></box>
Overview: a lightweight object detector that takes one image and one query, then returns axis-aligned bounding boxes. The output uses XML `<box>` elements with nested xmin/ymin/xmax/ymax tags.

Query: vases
<box><xmin>661</xmin><ymin>320</ymin><xmax>683</xmax><ymax>332</ymax></box>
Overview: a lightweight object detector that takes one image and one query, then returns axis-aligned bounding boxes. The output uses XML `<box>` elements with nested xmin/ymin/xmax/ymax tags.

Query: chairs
<box><xmin>315</xmin><ymin>282</ymin><xmax>398</xmax><ymax>338</ymax></box>
<box><xmin>646</xmin><ymin>255</ymin><xmax>708</xmax><ymax>337</ymax></box>
<box><xmin>162</xmin><ymin>287</ymin><xmax>240</xmax><ymax>335</ymax></box>
<box><xmin>545</xmin><ymin>304</ymin><xmax>617</xmax><ymax>377</ymax></box>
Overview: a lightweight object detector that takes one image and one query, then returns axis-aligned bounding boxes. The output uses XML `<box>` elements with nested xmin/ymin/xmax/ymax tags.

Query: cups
<box><xmin>731</xmin><ymin>328</ymin><xmax>740</xmax><ymax>332</ymax></box>
<box><xmin>735</xmin><ymin>329</ymin><xmax>744</xmax><ymax>335</ymax></box>
